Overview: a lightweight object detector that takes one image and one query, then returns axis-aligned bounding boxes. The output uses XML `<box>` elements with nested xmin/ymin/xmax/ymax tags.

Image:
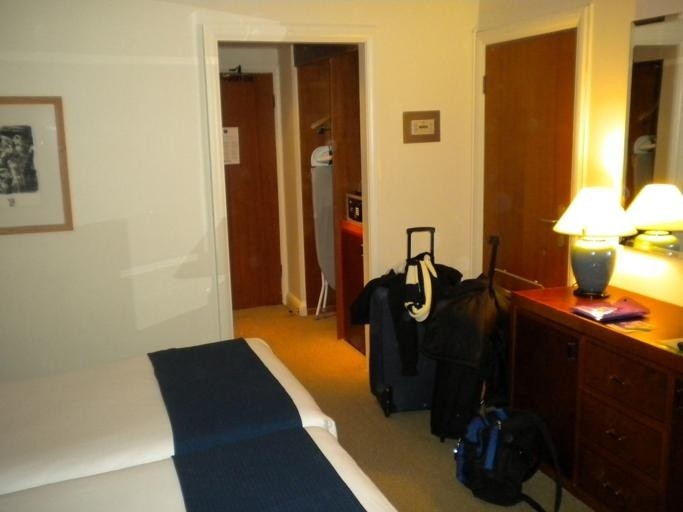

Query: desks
<box><xmin>508</xmin><ymin>286</ymin><xmax>682</xmax><ymax>511</ymax></box>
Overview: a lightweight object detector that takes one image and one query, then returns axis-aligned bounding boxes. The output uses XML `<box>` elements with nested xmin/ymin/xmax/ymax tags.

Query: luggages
<box><xmin>369</xmin><ymin>227</ymin><xmax>436</xmax><ymax>414</ymax></box>
<box><xmin>429</xmin><ymin>235</ymin><xmax>499</xmax><ymax>439</ymax></box>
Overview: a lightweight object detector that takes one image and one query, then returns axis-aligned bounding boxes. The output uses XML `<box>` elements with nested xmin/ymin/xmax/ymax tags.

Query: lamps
<box><xmin>552</xmin><ymin>186</ymin><xmax>637</xmax><ymax>297</ymax></box>
<box><xmin>626</xmin><ymin>183</ymin><xmax>683</xmax><ymax>252</ymax></box>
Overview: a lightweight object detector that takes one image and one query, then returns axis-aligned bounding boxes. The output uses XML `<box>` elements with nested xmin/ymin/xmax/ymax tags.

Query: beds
<box><xmin>0</xmin><ymin>427</ymin><xmax>399</xmax><ymax>512</ymax></box>
<box><xmin>1</xmin><ymin>335</ymin><xmax>338</xmax><ymax>493</ymax></box>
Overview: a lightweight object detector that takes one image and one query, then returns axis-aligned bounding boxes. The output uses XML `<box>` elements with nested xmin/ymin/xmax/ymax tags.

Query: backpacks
<box><xmin>455</xmin><ymin>409</ymin><xmax>561</xmax><ymax>510</ymax></box>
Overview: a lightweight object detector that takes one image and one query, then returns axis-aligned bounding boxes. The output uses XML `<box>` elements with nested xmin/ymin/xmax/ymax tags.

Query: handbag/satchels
<box><xmin>425</xmin><ymin>271</ymin><xmax>512</xmax><ymax>364</ymax></box>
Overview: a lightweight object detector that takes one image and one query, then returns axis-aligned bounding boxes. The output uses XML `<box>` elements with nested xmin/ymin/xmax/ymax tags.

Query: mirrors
<box><xmin>620</xmin><ymin>11</ymin><xmax>683</xmax><ymax>263</ymax></box>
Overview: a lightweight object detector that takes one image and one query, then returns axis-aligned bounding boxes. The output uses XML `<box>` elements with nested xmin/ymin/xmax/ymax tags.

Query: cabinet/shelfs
<box><xmin>334</xmin><ymin>221</ymin><xmax>365</xmax><ymax>353</ymax></box>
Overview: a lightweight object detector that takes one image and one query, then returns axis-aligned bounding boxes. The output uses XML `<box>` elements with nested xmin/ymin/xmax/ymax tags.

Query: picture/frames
<box><xmin>0</xmin><ymin>95</ymin><xmax>74</xmax><ymax>235</ymax></box>
